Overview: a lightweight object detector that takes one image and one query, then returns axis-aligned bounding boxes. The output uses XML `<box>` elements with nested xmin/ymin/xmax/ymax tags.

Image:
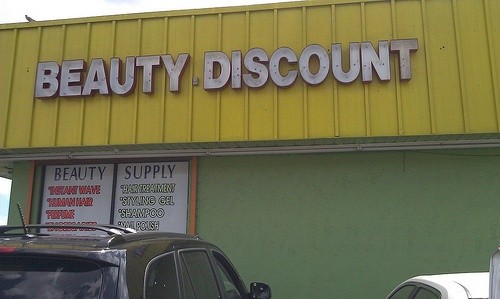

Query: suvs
<box><xmin>0</xmin><ymin>222</ymin><xmax>273</xmax><ymax>298</ymax></box>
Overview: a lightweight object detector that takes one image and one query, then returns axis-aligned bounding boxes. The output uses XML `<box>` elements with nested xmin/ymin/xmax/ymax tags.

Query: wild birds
<box><xmin>25</xmin><ymin>15</ymin><xmax>36</xmax><ymax>22</ymax></box>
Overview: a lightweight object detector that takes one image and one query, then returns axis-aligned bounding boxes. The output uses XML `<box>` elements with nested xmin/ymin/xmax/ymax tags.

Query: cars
<box><xmin>384</xmin><ymin>245</ymin><xmax>499</xmax><ymax>299</ymax></box>
